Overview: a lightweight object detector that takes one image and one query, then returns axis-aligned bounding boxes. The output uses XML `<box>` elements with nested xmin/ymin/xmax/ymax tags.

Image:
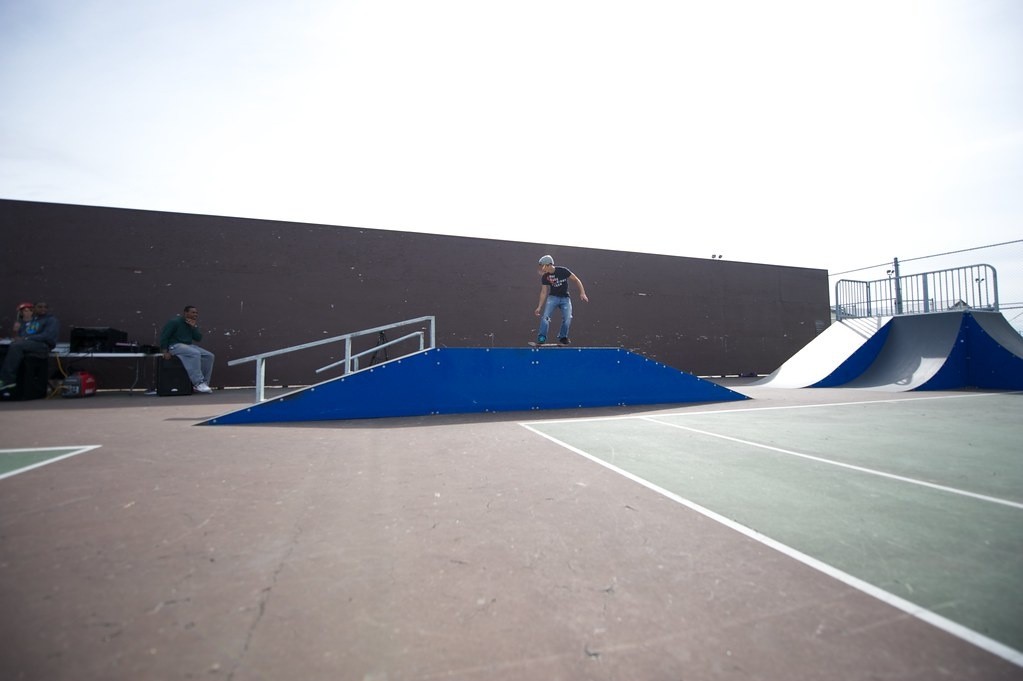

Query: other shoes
<box><xmin>0</xmin><ymin>380</ymin><xmax>16</xmax><ymax>391</ymax></box>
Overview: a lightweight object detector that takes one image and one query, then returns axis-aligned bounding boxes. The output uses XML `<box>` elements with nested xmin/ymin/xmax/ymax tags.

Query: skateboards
<box><xmin>527</xmin><ymin>340</ymin><xmax>571</xmax><ymax>348</ymax></box>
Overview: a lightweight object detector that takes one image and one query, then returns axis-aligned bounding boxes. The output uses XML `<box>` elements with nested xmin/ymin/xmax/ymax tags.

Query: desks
<box><xmin>45</xmin><ymin>352</ymin><xmax>164</xmax><ymax>396</ymax></box>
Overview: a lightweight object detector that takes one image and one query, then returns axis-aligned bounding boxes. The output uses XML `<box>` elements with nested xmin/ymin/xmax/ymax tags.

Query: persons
<box><xmin>0</xmin><ymin>295</ymin><xmax>59</xmax><ymax>391</ymax></box>
<box><xmin>158</xmin><ymin>305</ymin><xmax>215</xmax><ymax>394</ymax></box>
<box><xmin>534</xmin><ymin>255</ymin><xmax>589</xmax><ymax>343</ymax></box>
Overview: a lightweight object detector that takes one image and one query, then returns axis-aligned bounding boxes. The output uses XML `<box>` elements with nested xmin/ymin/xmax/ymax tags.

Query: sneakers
<box><xmin>560</xmin><ymin>337</ymin><xmax>571</xmax><ymax>345</ymax></box>
<box><xmin>537</xmin><ymin>335</ymin><xmax>546</xmax><ymax>344</ymax></box>
<box><xmin>193</xmin><ymin>383</ymin><xmax>212</xmax><ymax>394</ymax></box>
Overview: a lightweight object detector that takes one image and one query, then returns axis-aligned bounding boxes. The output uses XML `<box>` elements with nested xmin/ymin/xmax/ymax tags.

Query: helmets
<box><xmin>538</xmin><ymin>254</ymin><xmax>554</xmax><ymax>266</ymax></box>
